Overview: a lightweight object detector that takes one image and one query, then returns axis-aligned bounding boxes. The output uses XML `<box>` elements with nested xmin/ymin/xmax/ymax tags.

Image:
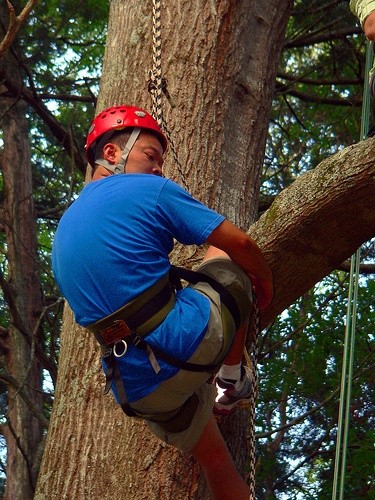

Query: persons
<box><xmin>52</xmin><ymin>104</ymin><xmax>275</xmax><ymax>500</ymax></box>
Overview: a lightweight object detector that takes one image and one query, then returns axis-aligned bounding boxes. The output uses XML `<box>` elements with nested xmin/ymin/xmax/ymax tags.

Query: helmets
<box><xmin>82</xmin><ymin>104</ymin><xmax>170</xmax><ymax>159</ymax></box>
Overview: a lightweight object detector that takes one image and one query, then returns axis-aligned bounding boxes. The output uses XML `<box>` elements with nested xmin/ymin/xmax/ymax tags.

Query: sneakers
<box><xmin>214</xmin><ymin>363</ymin><xmax>258</xmax><ymax>416</ymax></box>
